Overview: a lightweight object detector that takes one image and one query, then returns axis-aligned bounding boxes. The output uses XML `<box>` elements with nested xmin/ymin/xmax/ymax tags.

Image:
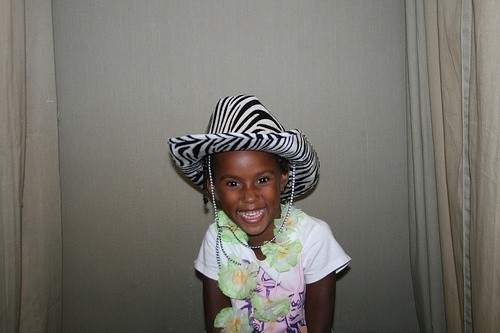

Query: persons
<box><xmin>168</xmin><ymin>93</ymin><xmax>351</xmax><ymax>333</ymax></box>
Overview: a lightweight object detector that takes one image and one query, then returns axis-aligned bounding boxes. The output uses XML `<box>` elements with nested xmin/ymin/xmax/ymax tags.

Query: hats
<box><xmin>166</xmin><ymin>94</ymin><xmax>321</xmax><ymax>202</ymax></box>
